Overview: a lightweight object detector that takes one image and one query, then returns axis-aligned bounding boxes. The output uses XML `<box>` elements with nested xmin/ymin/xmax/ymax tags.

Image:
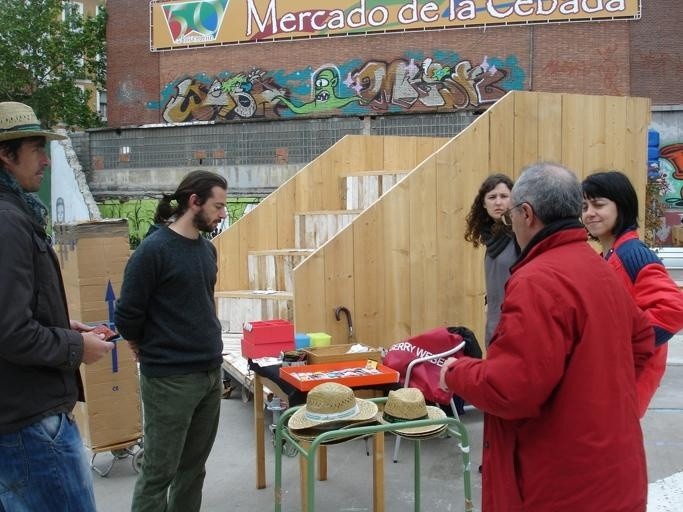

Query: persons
<box><xmin>462</xmin><ymin>171</ymin><xmax>520</xmax><ymax>353</ymax></box>
<box><xmin>578</xmin><ymin>169</ymin><xmax>682</xmax><ymax>421</ymax></box>
<box><xmin>0</xmin><ymin>99</ymin><xmax>119</xmax><ymax>512</ymax></box>
<box><xmin>436</xmin><ymin>160</ymin><xmax>656</xmax><ymax>510</ymax></box>
<box><xmin>110</xmin><ymin>170</ymin><xmax>227</xmax><ymax>511</ymax></box>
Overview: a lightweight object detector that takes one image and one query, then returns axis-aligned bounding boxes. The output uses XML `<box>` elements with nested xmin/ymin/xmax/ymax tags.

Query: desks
<box><xmin>247</xmin><ymin>357</ymin><xmax>402</xmax><ymax>512</ymax></box>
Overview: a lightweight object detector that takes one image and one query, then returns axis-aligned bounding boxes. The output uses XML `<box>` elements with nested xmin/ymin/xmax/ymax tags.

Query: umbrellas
<box><xmin>335</xmin><ymin>306</ymin><xmax>372</xmax><ymax>456</ymax></box>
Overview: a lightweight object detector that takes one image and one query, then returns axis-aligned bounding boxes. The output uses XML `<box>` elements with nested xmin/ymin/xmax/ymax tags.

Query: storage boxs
<box><xmin>51</xmin><ymin>217</ymin><xmax>144</xmax><ymax>449</ymax></box>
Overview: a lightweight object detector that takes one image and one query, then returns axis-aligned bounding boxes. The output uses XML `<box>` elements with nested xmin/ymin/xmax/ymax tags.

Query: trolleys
<box><xmin>89</xmin><ymin>437</ymin><xmax>146</xmax><ymax>477</ymax></box>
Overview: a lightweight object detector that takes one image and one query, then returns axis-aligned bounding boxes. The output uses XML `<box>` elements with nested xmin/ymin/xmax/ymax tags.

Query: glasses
<box><xmin>501</xmin><ymin>204</ymin><xmax>524</xmax><ymax>225</ymax></box>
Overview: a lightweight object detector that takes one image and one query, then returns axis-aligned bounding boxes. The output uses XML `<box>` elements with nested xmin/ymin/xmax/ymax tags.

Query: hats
<box><xmin>0</xmin><ymin>102</ymin><xmax>68</xmax><ymax>142</ymax></box>
<box><xmin>288</xmin><ymin>382</ymin><xmax>448</xmax><ymax>446</ymax></box>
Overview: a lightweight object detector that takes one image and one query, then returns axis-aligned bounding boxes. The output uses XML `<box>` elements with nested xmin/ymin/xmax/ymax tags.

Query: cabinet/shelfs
<box><xmin>274</xmin><ymin>396</ymin><xmax>474</xmax><ymax>511</ymax></box>
